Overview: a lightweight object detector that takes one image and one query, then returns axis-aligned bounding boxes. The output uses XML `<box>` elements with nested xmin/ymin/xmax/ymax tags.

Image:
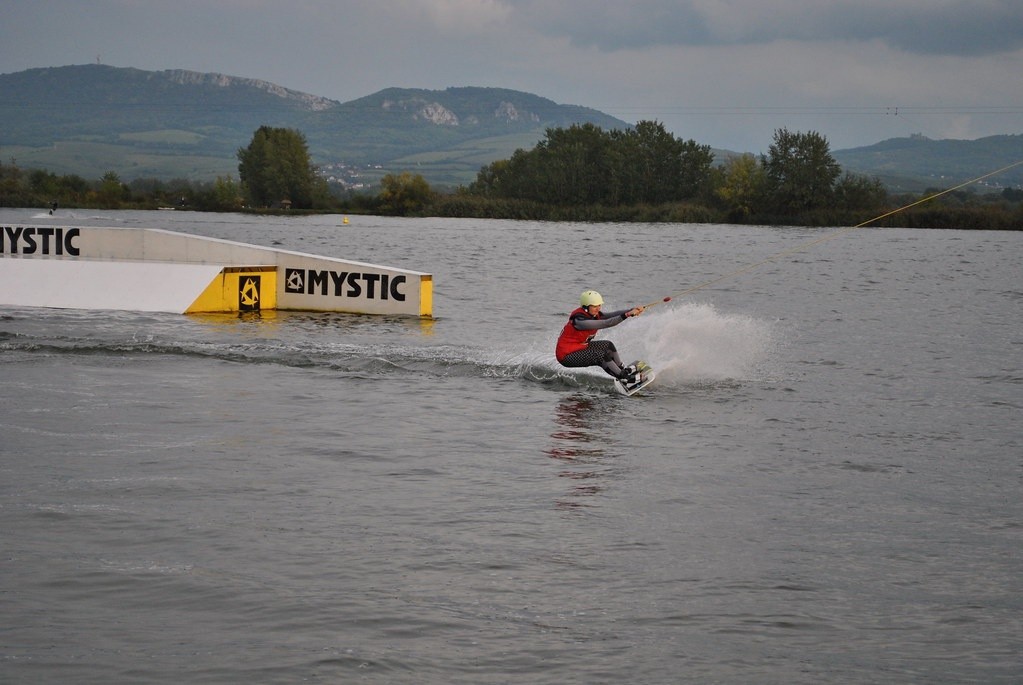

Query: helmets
<box><xmin>579</xmin><ymin>291</ymin><xmax>604</xmax><ymax>309</ymax></box>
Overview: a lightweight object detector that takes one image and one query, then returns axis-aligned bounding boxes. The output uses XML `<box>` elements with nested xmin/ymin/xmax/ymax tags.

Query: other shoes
<box><xmin>624</xmin><ymin>373</ymin><xmax>644</xmax><ymax>391</ymax></box>
<box><xmin>624</xmin><ymin>364</ymin><xmax>639</xmax><ymax>376</ymax></box>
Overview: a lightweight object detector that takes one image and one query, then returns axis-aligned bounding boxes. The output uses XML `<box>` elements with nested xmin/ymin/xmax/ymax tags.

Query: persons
<box><xmin>556</xmin><ymin>290</ymin><xmax>646</xmax><ymax>390</ymax></box>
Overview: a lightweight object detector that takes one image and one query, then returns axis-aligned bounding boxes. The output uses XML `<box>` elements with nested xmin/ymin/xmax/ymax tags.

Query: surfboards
<box><xmin>615</xmin><ymin>361</ymin><xmax>656</xmax><ymax>397</ymax></box>
